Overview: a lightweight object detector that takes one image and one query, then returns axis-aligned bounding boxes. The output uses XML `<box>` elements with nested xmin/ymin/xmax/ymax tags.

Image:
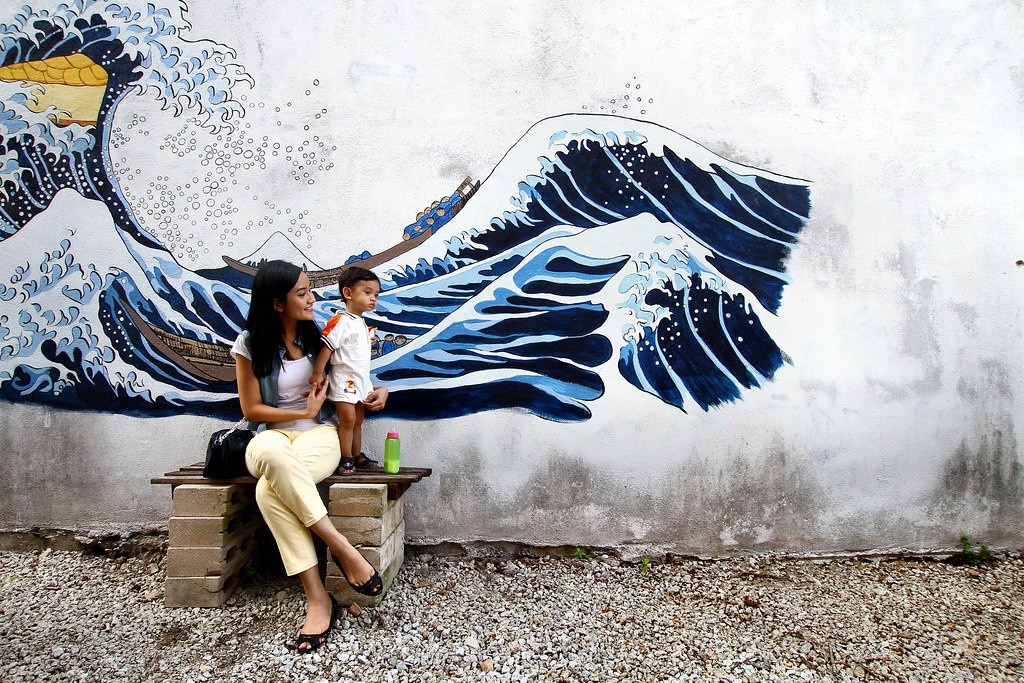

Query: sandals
<box><xmin>331</xmin><ymin>546</ymin><xmax>383</xmax><ymax>596</ymax></box>
<box><xmin>338</xmin><ymin>457</ymin><xmax>356</xmax><ymax>475</ymax></box>
<box><xmin>353</xmin><ymin>452</ymin><xmax>379</xmax><ymax>469</ymax></box>
<box><xmin>295</xmin><ymin>592</ymin><xmax>339</xmax><ymax>652</ymax></box>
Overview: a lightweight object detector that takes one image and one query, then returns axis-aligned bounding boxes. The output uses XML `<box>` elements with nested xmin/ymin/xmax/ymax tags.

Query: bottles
<box><xmin>383</xmin><ymin>424</ymin><xmax>401</xmax><ymax>474</ymax></box>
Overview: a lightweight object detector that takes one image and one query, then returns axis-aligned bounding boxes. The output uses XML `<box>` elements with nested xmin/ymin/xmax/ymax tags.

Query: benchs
<box><xmin>150</xmin><ymin>462</ymin><xmax>432</xmax><ymax>606</ymax></box>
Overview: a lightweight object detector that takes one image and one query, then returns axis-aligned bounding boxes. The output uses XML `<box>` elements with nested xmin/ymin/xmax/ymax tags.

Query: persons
<box><xmin>308</xmin><ymin>267</ymin><xmax>380</xmax><ymax>477</ymax></box>
<box><xmin>229</xmin><ymin>260</ymin><xmax>388</xmax><ymax>652</ymax></box>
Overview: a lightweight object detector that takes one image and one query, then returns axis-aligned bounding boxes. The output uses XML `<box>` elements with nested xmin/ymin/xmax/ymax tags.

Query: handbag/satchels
<box><xmin>203</xmin><ymin>429</ymin><xmax>254</xmax><ymax>480</ymax></box>
